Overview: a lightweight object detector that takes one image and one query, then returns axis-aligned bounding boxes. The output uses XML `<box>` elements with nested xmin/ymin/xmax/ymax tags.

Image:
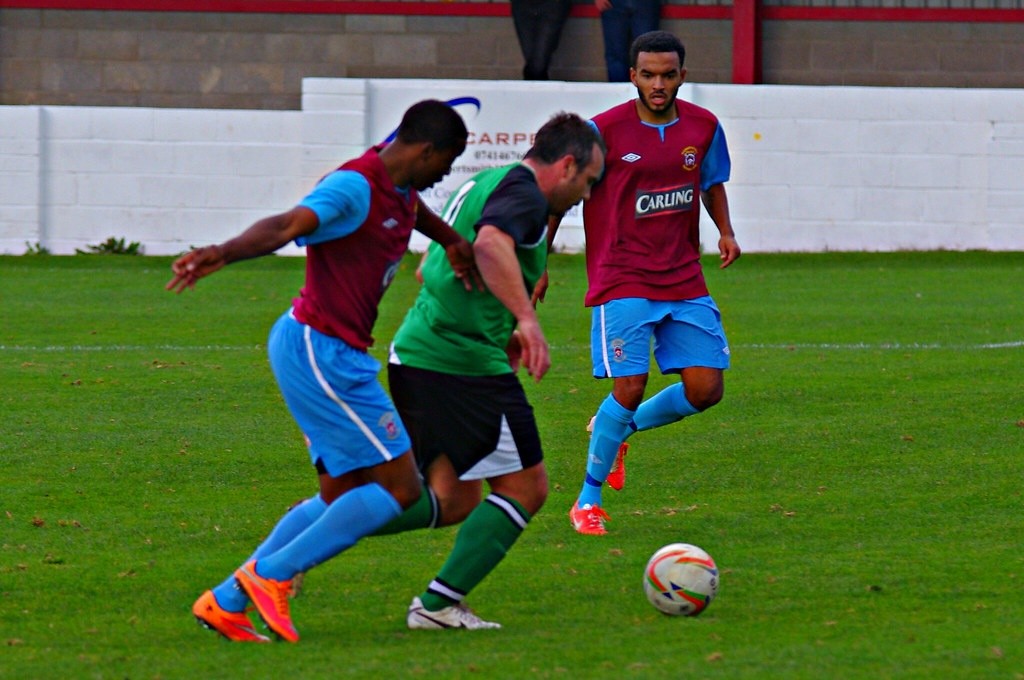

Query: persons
<box><xmin>510</xmin><ymin>0</ymin><xmax>592</xmax><ymax>80</ymax></box>
<box><xmin>528</xmin><ymin>30</ymin><xmax>740</xmax><ymax>534</ymax></box>
<box><xmin>290</xmin><ymin>114</ymin><xmax>605</xmax><ymax>631</ymax></box>
<box><xmin>165</xmin><ymin>100</ymin><xmax>485</xmax><ymax>644</ymax></box>
<box><xmin>595</xmin><ymin>0</ymin><xmax>667</xmax><ymax>82</ymax></box>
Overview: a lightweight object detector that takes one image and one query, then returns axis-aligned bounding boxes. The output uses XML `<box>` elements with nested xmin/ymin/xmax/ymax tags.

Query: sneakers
<box><xmin>586</xmin><ymin>415</ymin><xmax>629</xmax><ymax>489</ymax></box>
<box><xmin>192</xmin><ymin>591</ymin><xmax>271</xmax><ymax>642</ymax></box>
<box><xmin>569</xmin><ymin>501</ymin><xmax>611</xmax><ymax>535</ymax></box>
<box><xmin>408</xmin><ymin>595</ymin><xmax>503</xmax><ymax>627</ymax></box>
<box><xmin>234</xmin><ymin>559</ymin><xmax>299</xmax><ymax>643</ymax></box>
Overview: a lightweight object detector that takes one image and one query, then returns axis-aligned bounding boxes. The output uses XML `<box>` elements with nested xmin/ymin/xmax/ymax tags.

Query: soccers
<box><xmin>643</xmin><ymin>542</ymin><xmax>720</xmax><ymax>616</ymax></box>
